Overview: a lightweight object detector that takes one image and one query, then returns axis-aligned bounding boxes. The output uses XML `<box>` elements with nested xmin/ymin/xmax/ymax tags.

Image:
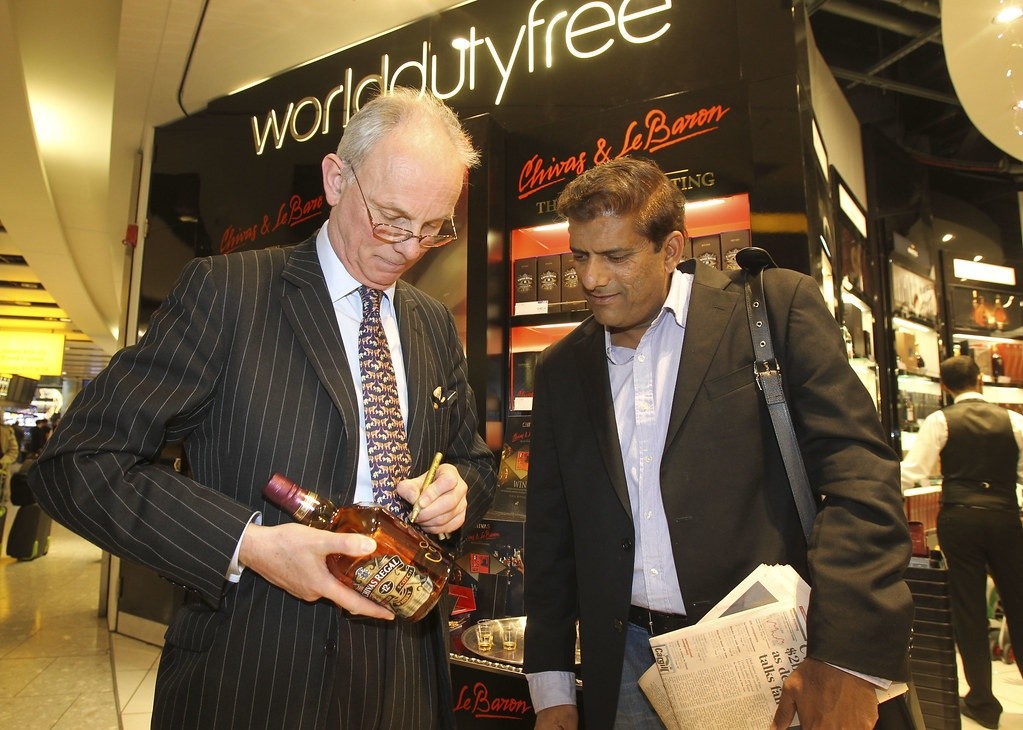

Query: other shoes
<box><xmin>959</xmin><ymin>695</ymin><xmax>1002</xmax><ymax>730</ymax></box>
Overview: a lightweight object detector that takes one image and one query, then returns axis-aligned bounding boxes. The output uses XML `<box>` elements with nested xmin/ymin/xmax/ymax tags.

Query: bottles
<box><xmin>990</xmin><ymin>344</ymin><xmax>1004</xmax><ymax>384</ymax></box>
<box><xmin>992</xmin><ymin>294</ymin><xmax>1008</xmax><ymax>330</ymax></box>
<box><xmin>913</xmin><ymin>344</ymin><xmax>925</xmax><ymax>367</ymax></box>
<box><xmin>897</xmin><ymin>393</ymin><xmax>919</xmax><ymax>432</ymax></box>
<box><xmin>971</xmin><ymin>290</ymin><xmax>991</xmax><ymax>327</ymax></box>
<box><xmin>263</xmin><ymin>472</ymin><xmax>453</xmax><ymax>624</ymax></box>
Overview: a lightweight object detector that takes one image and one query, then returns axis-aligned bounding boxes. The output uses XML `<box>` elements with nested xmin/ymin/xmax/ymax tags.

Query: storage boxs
<box><xmin>722</xmin><ymin>229</ymin><xmax>749</xmax><ymax>272</ymax></box>
<box><xmin>515</xmin><ymin>256</ymin><xmax>538</xmax><ymax>304</ymax></box>
<box><xmin>537</xmin><ymin>254</ymin><xmax>561</xmax><ymax>312</ymax></box>
<box><xmin>561</xmin><ymin>253</ymin><xmax>587</xmax><ymax>313</ymax></box>
<box><xmin>513</xmin><ymin>351</ymin><xmax>544</xmax><ymax>410</ymax></box>
<box><xmin>681</xmin><ymin>236</ymin><xmax>693</xmax><ymax>262</ymax></box>
<box><xmin>693</xmin><ymin>233</ymin><xmax>722</xmax><ymax>271</ymax></box>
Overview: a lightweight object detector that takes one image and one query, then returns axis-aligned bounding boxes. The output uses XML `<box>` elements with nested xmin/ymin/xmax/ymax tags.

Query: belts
<box><xmin>627</xmin><ymin>604</ymin><xmax>688</xmax><ymax>637</ymax></box>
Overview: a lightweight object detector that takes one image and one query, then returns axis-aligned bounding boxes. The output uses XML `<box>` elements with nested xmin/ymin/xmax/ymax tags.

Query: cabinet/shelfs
<box><xmin>492</xmin><ymin>76</ymin><xmax>1023</xmax><ymax>515</ymax></box>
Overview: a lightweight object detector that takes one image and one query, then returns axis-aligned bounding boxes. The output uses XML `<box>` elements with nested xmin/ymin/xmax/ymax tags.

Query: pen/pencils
<box><xmin>411</xmin><ymin>452</ymin><xmax>443</xmax><ymax>523</ymax></box>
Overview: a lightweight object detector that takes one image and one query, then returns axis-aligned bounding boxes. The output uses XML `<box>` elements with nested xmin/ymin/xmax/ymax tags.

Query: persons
<box><xmin>0</xmin><ymin>406</ymin><xmax>62</xmax><ymax>545</ymax></box>
<box><xmin>520</xmin><ymin>156</ymin><xmax>916</xmax><ymax>730</ymax></box>
<box><xmin>900</xmin><ymin>355</ymin><xmax>1023</xmax><ymax>730</ymax></box>
<box><xmin>26</xmin><ymin>84</ymin><xmax>499</xmax><ymax>730</ymax></box>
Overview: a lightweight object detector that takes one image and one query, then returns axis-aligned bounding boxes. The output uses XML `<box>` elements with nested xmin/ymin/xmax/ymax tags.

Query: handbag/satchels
<box><xmin>10</xmin><ymin>458</ymin><xmax>37</xmax><ymax>507</ymax></box>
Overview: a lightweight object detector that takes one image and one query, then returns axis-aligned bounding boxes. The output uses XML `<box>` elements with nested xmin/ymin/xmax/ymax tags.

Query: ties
<box><xmin>356</xmin><ymin>285</ymin><xmax>414</xmax><ymax>524</ymax></box>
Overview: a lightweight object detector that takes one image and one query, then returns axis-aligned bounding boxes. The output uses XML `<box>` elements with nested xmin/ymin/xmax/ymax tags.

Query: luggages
<box><xmin>6</xmin><ymin>506</ymin><xmax>51</xmax><ymax>562</ymax></box>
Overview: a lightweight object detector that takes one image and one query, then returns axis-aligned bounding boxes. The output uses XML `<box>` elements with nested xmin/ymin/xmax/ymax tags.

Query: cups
<box><xmin>475</xmin><ymin>627</ymin><xmax>493</xmax><ymax>651</ymax></box>
<box><xmin>502</xmin><ymin>626</ymin><xmax>517</xmax><ymax>651</ymax></box>
<box><xmin>478</xmin><ymin>618</ymin><xmax>493</xmax><ymax>634</ymax></box>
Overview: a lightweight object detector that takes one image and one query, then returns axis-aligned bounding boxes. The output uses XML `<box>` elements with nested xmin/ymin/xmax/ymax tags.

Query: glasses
<box><xmin>348</xmin><ymin>159</ymin><xmax>458</xmax><ymax>248</ymax></box>
